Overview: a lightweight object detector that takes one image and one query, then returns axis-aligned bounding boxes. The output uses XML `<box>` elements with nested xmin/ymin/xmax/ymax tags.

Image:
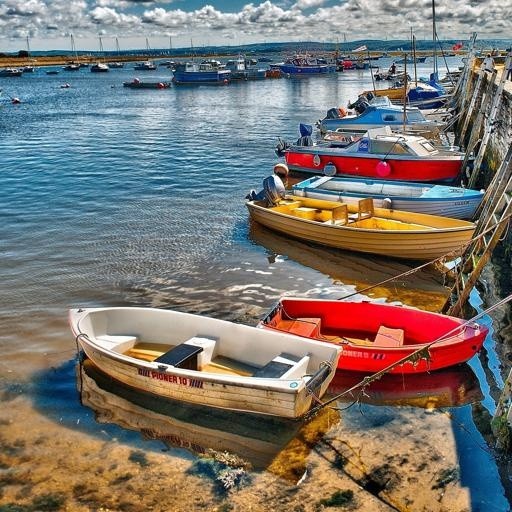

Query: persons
<box><xmin>483</xmin><ymin>53</ymin><xmax>495</xmax><ymax>73</ymax></box>
<box><xmin>390</xmin><ymin>62</ymin><xmax>397</xmax><ymax>75</ymax></box>
<box><xmin>504</xmin><ymin>46</ymin><xmax>512</xmax><ymax>82</ymax></box>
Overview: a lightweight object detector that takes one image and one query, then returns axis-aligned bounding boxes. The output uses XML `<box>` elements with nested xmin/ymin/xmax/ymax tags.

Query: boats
<box><xmin>122</xmin><ymin>78</ymin><xmax>171</xmax><ymax>89</ymax></box>
<box><xmin>244</xmin><ymin>124</ymin><xmax>486</xmax><ymax>263</ymax></box>
<box><xmin>254</xmin><ymin>296</ymin><xmax>489</xmax><ymax>375</ymax></box>
<box><xmin>67</xmin><ymin>307</ymin><xmax>343</xmax><ymax>420</ymax></box>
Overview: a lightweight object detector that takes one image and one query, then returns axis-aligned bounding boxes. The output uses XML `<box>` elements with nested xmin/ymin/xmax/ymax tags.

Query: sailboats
<box><xmin>0</xmin><ymin>35</ymin><xmax>35</xmax><ymax>77</ymax></box>
<box><xmin>159</xmin><ymin>34</ymin><xmax>386</xmax><ymax>86</ymax></box>
<box><xmin>62</xmin><ymin>34</ymin><xmax>124</xmax><ymax>72</ymax></box>
<box><xmin>316</xmin><ymin>0</ymin><xmax>459</xmax><ymax>132</ymax></box>
<box><xmin>132</xmin><ymin>36</ymin><xmax>157</xmax><ymax>71</ymax></box>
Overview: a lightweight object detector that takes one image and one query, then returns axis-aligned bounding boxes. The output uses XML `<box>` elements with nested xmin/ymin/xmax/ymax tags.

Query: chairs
<box><xmin>325</xmin><ymin>205</ymin><xmax>348</xmax><ymax>226</ymax></box>
<box><xmin>348</xmin><ymin>197</ymin><xmax>374</xmax><ymax>222</ymax></box>
